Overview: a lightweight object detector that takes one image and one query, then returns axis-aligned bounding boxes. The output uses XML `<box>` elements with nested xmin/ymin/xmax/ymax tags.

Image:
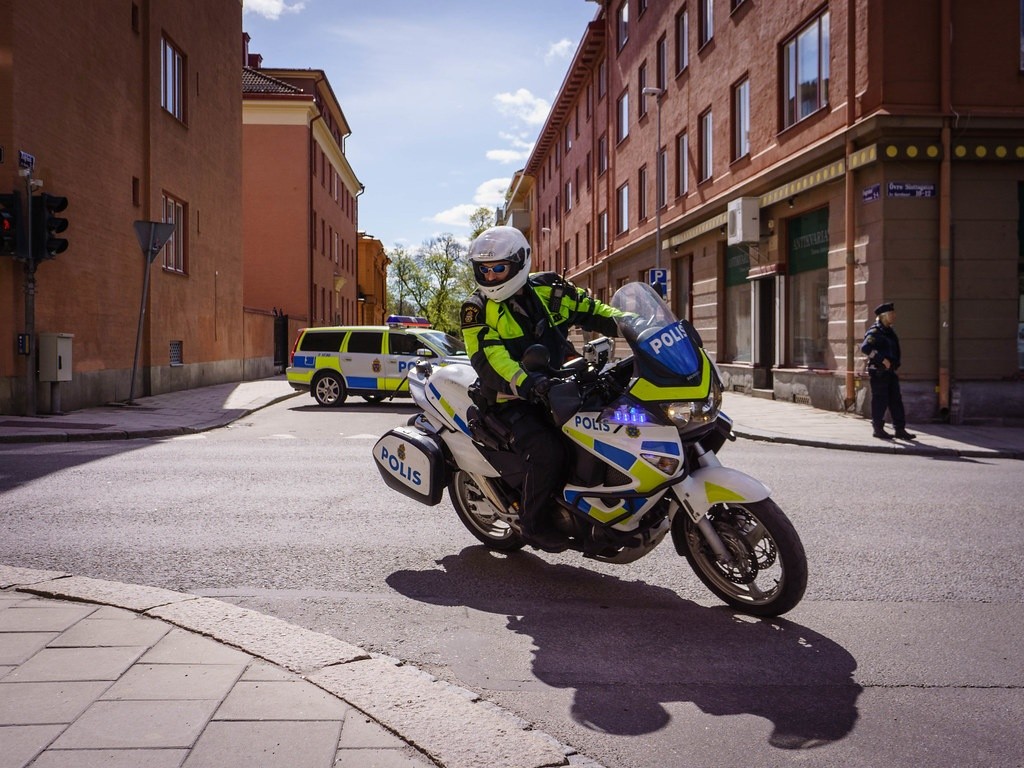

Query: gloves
<box><xmin>518</xmin><ymin>371</ymin><xmax>550</xmax><ymax>412</ymax></box>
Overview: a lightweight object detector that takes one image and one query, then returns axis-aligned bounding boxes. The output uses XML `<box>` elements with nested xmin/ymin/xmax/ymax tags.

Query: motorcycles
<box><xmin>372</xmin><ymin>281</ymin><xmax>809</xmax><ymax>619</ymax></box>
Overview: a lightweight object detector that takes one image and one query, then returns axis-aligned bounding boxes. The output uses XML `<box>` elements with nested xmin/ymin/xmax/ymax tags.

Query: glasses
<box><xmin>478</xmin><ymin>264</ymin><xmax>510</xmax><ymax>274</ymax></box>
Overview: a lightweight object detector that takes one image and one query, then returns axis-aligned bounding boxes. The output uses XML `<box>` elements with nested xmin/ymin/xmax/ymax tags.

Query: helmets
<box><xmin>467</xmin><ymin>225</ymin><xmax>532</xmax><ymax>303</ymax></box>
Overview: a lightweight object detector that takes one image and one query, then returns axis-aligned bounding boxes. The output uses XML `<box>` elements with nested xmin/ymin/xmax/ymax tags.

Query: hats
<box><xmin>873</xmin><ymin>303</ymin><xmax>895</xmax><ymax>315</ymax></box>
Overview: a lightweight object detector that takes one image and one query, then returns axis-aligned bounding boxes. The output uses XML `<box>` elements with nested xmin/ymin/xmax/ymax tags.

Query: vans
<box><xmin>285</xmin><ymin>314</ymin><xmax>473</xmax><ymax>407</ymax></box>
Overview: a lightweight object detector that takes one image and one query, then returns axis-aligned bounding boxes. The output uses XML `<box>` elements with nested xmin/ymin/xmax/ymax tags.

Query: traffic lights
<box><xmin>0</xmin><ymin>191</ymin><xmax>25</xmax><ymax>258</ymax></box>
<box><xmin>40</xmin><ymin>192</ymin><xmax>68</xmax><ymax>260</ymax></box>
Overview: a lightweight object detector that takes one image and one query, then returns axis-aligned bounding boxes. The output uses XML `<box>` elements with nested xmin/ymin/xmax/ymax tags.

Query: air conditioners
<box><xmin>728</xmin><ymin>197</ymin><xmax>760</xmax><ymax>245</ymax></box>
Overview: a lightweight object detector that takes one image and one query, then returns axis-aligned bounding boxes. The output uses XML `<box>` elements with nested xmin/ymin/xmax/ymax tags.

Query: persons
<box><xmin>861</xmin><ymin>302</ymin><xmax>917</xmax><ymax>439</ymax></box>
<box><xmin>460</xmin><ymin>225</ymin><xmax>650</xmax><ymax>552</ymax></box>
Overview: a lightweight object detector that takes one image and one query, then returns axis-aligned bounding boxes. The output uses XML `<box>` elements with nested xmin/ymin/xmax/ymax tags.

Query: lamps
<box><xmin>642</xmin><ymin>87</ymin><xmax>661</xmax><ymax>95</ymax></box>
<box><xmin>675</xmin><ymin>247</ymin><xmax>679</xmax><ymax>253</ymax></box>
<box><xmin>721</xmin><ymin>228</ymin><xmax>725</xmax><ymax>234</ymax></box>
<box><xmin>789</xmin><ymin>197</ymin><xmax>796</xmax><ymax>209</ymax></box>
<box><xmin>358</xmin><ymin>293</ymin><xmax>365</xmax><ymax>301</ymax></box>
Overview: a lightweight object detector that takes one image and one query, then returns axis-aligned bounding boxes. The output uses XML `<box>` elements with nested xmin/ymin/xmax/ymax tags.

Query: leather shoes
<box><xmin>519</xmin><ymin>523</ymin><xmax>570</xmax><ymax>553</ymax></box>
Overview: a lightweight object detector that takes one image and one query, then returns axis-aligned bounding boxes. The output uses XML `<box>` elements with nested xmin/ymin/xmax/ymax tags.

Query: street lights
<box><xmin>642</xmin><ymin>87</ymin><xmax>663</xmax><ymax>268</ymax></box>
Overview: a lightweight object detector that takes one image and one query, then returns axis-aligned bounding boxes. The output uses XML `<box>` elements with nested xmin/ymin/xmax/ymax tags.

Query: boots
<box><xmin>893</xmin><ymin>427</ymin><xmax>916</xmax><ymax>440</ymax></box>
<box><xmin>870</xmin><ymin>419</ymin><xmax>895</xmax><ymax>439</ymax></box>
